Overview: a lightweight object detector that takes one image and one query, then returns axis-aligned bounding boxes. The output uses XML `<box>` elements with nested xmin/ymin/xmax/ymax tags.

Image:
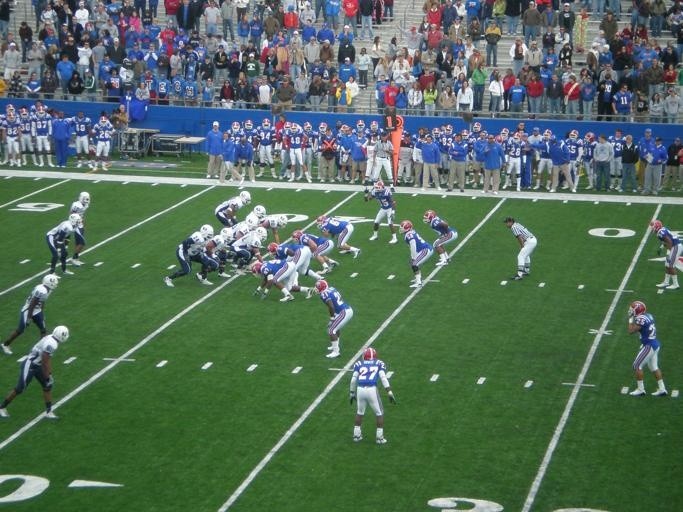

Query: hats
<box><xmin>503</xmin><ymin>216</ymin><xmax>514</xmax><ymax>223</ymax></box>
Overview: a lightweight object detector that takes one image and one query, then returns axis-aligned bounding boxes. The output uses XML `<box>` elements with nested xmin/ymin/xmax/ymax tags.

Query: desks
<box><xmin>170</xmin><ymin>134</ymin><xmax>208</xmax><ymax>161</ymax></box>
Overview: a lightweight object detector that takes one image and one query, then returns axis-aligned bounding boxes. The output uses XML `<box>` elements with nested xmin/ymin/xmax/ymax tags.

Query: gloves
<box><xmin>26</xmin><ymin>319</ymin><xmax>31</xmax><ymax>327</ymax></box>
<box><xmin>46</xmin><ymin>375</ymin><xmax>54</xmax><ymax>387</ymax></box>
<box><xmin>656</xmin><ymin>247</ymin><xmax>661</xmax><ymax>256</ymax></box>
<box><xmin>388</xmin><ymin>393</ymin><xmax>396</xmax><ymax>404</ymax></box>
<box><xmin>349</xmin><ymin>391</ymin><xmax>357</xmax><ymax>405</ymax></box>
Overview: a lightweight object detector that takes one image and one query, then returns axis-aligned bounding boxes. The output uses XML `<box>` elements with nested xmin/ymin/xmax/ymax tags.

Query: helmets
<box><xmin>628</xmin><ymin>301</ymin><xmax>646</xmax><ymax>317</ymax></box>
<box><xmin>79</xmin><ymin>192</ymin><xmax>90</xmax><ymax>205</ymax></box>
<box><xmin>431</xmin><ymin>122</ymin><xmax>521</xmax><ymax>146</ymax></box>
<box><xmin>314</xmin><ymin>280</ymin><xmax>328</xmax><ymax>294</ymax></box>
<box><xmin>649</xmin><ymin>220</ymin><xmax>661</xmax><ymax>233</ymax></box>
<box><xmin>316</xmin><ymin>215</ymin><xmax>327</xmax><ymax>225</ymax></box>
<box><xmin>201</xmin><ymin>190</ymin><xmax>288</xmax><ymax>242</ymax></box>
<box><xmin>41</xmin><ymin>274</ymin><xmax>58</xmax><ymax>290</ymax></box>
<box><xmin>542</xmin><ymin>129</ymin><xmax>594</xmax><ymax>143</ymax></box>
<box><xmin>373</xmin><ymin>181</ymin><xmax>385</xmax><ymax>193</ymax></box>
<box><xmin>100</xmin><ymin>116</ymin><xmax>106</xmax><ymax>124</ymax></box>
<box><xmin>398</xmin><ymin>220</ymin><xmax>412</xmax><ymax>235</ymax></box>
<box><xmin>362</xmin><ymin>348</ymin><xmax>376</xmax><ymax>360</ymax></box>
<box><xmin>232</xmin><ymin>118</ymin><xmax>377</xmax><ymax>132</ymax></box>
<box><xmin>252</xmin><ymin>262</ymin><xmax>262</xmax><ymax>274</ymax></box>
<box><xmin>5</xmin><ymin>103</ymin><xmax>46</xmax><ymax>120</ymax></box>
<box><xmin>292</xmin><ymin>230</ymin><xmax>302</xmax><ymax>242</ymax></box>
<box><xmin>52</xmin><ymin>325</ymin><xmax>69</xmax><ymax>343</ymax></box>
<box><xmin>422</xmin><ymin>210</ymin><xmax>436</xmax><ymax>223</ymax></box>
<box><xmin>68</xmin><ymin>213</ymin><xmax>82</xmax><ymax>227</ymax></box>
<box><xmin>267</xmin><ymin>243</ymin><xmax>279</xmax><ymax>254</ymax></box>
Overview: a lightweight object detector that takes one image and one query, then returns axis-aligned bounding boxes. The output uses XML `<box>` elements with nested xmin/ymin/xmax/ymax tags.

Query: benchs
<box><xmin>1</xmin><ymin>0</ymin><xmax>682</xmax><ymax>122</ymax></box>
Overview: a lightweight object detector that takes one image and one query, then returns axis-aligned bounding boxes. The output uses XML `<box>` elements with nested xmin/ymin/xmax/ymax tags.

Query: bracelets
<box><xmin>628</xmin><ymin>319</ymin><xmax>635</xmax><ymax>324</ymax></box>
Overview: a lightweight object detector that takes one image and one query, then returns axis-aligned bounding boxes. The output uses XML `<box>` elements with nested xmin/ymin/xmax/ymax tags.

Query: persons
<box><xmin>651</xmin><ymin>220</ymin><xmax>683</xmax><ymax>289</ymax></box>
<box><xmin>348</xmin><ymin>348</ymin><xmax>396</xmax><ymax>443</ymax></box>
<box><xmin>423</xmin><ymin>210</ymin><xmax>457</xmax><ymax>265</ymax></box>
<box><xmin>0</xmin><ymin>325</ymin><xmax>69</xmax><ymax>419</ymax></box>
<box><xmin>0</xmin><ymin>1</ymin><xmax>375</xmax><ymax>113</ymax></box>
<box><xmin>0</xmin><ymin>102</ymin><xmax>128</xmax><ymax>173</ymax></box>
<box><xmin>0</xmin><ymin>274</ymin><xmax>59</xmax><ymax>355</ymax></box>
<box><xmin>364</xmin><ymin>181</ymin><xmax>398</xmax><ymax>243</ymax></box>
<box><xmin>628</xmin><ymin>300</ymin><xmax>668</xmax><ymax>397</ymax></box>
<box><xmin>206</xmin><ymin>122</ymin><xmax>683</xmax><ymax>195</ymax></box>
<box><xmin>399</xmin><ymin>220</ymin><xmax>433</xmax><ymax>287</ymax></box>
<box><xmin>504</xmin><ymin>216</ymin><xmax>537</xmax><ymax>280</ymax></box>
<box><xmin>164</xmin><ymin>191</ymin><xmax>360</xmax><ymax>301</ymax></box>
<box><xmin>46</xmin><ymin>192</ymin><xmax>90</xmax><ymax>274</ymax></box>
<box><xmin>315</xmin><ymin>280</ymin><xmax>353</xmax><ymax>359</ymax></box>
<box><xmin>369</xmin><ymin>0</ymin><xmax>683</xmax><ymax>123</ymax></box>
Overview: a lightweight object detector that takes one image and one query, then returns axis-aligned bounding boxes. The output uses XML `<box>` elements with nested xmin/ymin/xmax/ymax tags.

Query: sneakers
<box><xmin>650</xmin><ymin>387</ymin><xmax>667</xmax><ymax>396</ymax></box>
<box><xmin>629</xmin><ymin>388</ymin><xmax>646</xmax><ymax>396</ymax></box>
<box><xmin>305</xmin><ymin>287</ymin><xmax>315</xmax><ymax>299</ymax></box>
<box><xmin>410</xmin><ymin>278</ymin><xmax>422</xmax><ymax>282</ymax></box>
<box><xmin>389</xmin><ymin>238</ymin><xmax>398</xmax><ymax>244</ymax></box>
<box><xmin>206</xmin><ymin>172</ymin><xmax>679</xmax><ymax>195</ymax></box>
<box><xmin>317</xmin><ymin>248</ymin><xmax>360</xmax><ymax>280</ymax></box>
<box><xmin>327</xmin><ymin>346</ymin><xmax>338</xmax><ymax>351</ymax></box>
<box><xmin>51</xmin><ymin>259</ymin><xmax>84</xmax><ymax>279</ymax></box>
<box><xmin>369</xmin><ymin>235</ymin><xmax>378</xmax><ymax>240</ymax></box>
<box><xmin>352</xmin><ymin>437</ymin><xmax>363</xmax><ymax>442</ymax></box>
<box><xmin>163</xmin><ymin>276</ymin><xmax>175</xmax><ymax>287</ymax></box>
<box><xmin>436</xmin><ymin>260</ymin><xmax>448</xmax><ymax>266</ymax></box>
<box><xmin>3</xmin><ymin>159</ymin><xmax>108</xmax><ymax>171</ymax></box>
<box><xmin>326</xmin><ymin>351</ymin><xmax>340</xmax><ymax>358</ymax></box>
<box><xmin>196</xmin><ymin>257</ymin><xmax>252</xmax><ymax>285</ymax></box>
<box><xmin>439</xmin><ymin>257</ymin><xmax>449</xmax><ymax>262</ymax></box>
<box><xmin>45</xmin><ymin>410</ymin><xmax>60</xmax><ymax>419</ymax></box>
<box><xmin>511</xmin><ymin>274</ymin><xmax>522</xmax><ymax>280</ymax></box>
<box><xmin>409</xmin><ymin>282</ymin><xmax>422</xmax><ymax>288</ymax></box>
<box><xmin>0</xmin><ymin>407</ymin><xmax>11</xmax><ymax>417</ymax></box>
<box><xmin>375</xmin><ymin>437</ymin><xmax>386</xmax><ymax>444</ymax></box>
<box><xmin>522</xmin><ymin>270</ymin><xmax>529</xmax><ymax>275</ymax></box>
<box><xmin>655</xmin><ymin>282</ymin><xmax>669</xmax><ymax>288</ymax></box>
<box><xmin>279</xmin><ymin>294</ymin><xmax>295</xmax><ymax>302</ymax></box>
<box><xmin>666</xmin><ymin>284</ymin><xmax>679</xmax><ymax>289</ymax></box>
<box><xmin>1</xmin><ymin>344</ymin><xmax>14</xmax><ymax>354</ymax></box>
